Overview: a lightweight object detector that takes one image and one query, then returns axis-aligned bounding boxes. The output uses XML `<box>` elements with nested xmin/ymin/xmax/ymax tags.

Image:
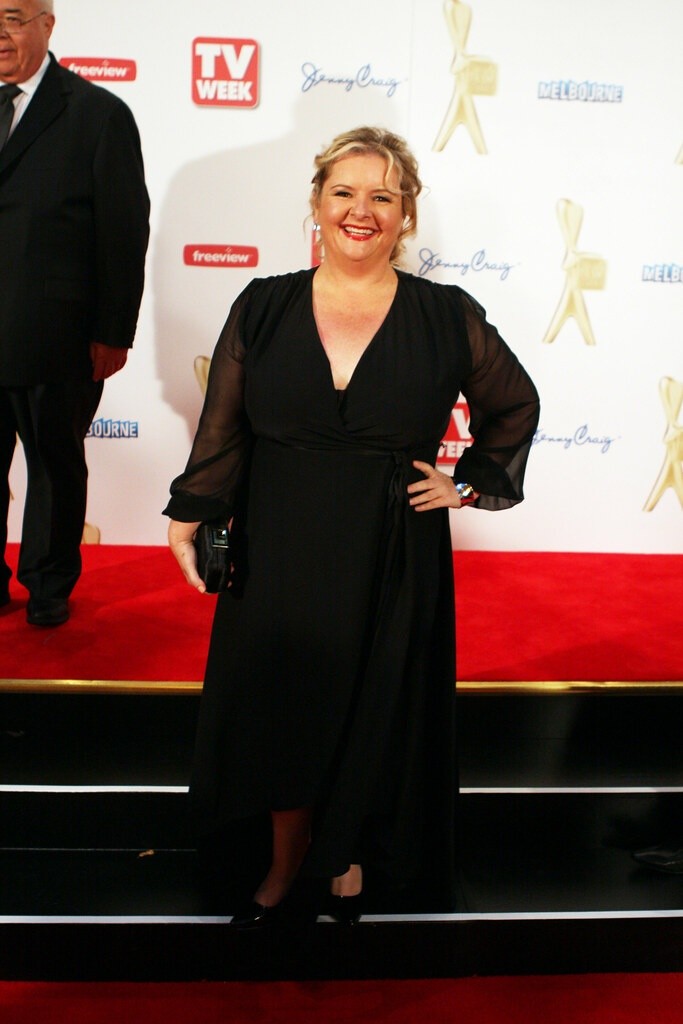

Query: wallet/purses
<box><xmin>197</xmin><ymin>523</ymin><xmax>231</xmax><ymax>593</ymax></box>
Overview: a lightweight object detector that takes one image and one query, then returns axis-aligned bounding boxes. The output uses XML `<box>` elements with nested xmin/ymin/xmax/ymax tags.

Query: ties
<box><xmin>0</xmin><ymin>85</ymin><xmax>23</xmax><ymax>154</ymax></box>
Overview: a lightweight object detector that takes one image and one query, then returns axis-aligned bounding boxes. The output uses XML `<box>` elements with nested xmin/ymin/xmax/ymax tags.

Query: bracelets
<box><xmin>451</xmin><ymin>476</ymin><xmax>474</xmax><ymax>508</ymax></box>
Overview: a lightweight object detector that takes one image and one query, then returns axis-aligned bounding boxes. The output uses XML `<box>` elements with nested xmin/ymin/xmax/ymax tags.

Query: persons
<box><xmin>0</xmin><ymin>0</ymin><xmax>151</xmax><ymax>626</ymax></box>
<box><xmin>162</xmin><ymin>126</ymin><xmax>540</xmax><ymax>932</ymax></box>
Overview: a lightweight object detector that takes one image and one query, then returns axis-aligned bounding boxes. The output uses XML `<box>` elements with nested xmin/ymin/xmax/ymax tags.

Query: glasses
<box><xmin>0</xmin><ymin>10</ymin><xmax>46</xmax><ymax>35</ymax></box>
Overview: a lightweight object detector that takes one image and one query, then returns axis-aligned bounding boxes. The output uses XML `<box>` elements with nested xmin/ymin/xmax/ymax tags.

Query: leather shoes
<box><xmin>26</xmin><ymin>597</ymin><xmax>68</xmax><ymax>625</ymax></box>
<box><xmin>631</xmin><ymin>842</ymin><xmax>683</xmax><ymax>874</ymax></box>
<box><xmin>328</xmin><ymin>888</ymin><xmax>366</xmax><ymax>931</ymax></box>
<box><xmin>0</xmin><ymin>584</ymin><xmax>10</xmax><ymax>608</ymax></box>
<box><xmin>229</xmin><ymin>893</ymin><xmax>292</xmax><ymax>929</ymax></box>
